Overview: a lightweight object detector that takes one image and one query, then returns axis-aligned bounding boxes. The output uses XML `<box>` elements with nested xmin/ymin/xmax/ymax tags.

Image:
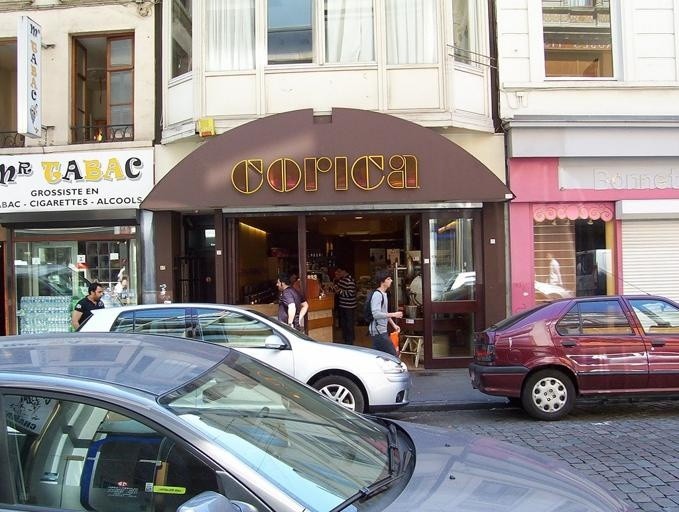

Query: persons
<box><xmin>363</xmin><ymin>272</ymin><xmax>403</xmax><ymax>356</ymax></box>
<box><xmin>333</xmin><ymin>262</ymin><xmax>358</xmax><ymax>345</ymax></box>
<box><xmin>408</xmin><ymin>261</ymin><xmax>443</xmax><ymax>306</ymax></box>
<box><xmin>276</xmin><ymin>273</ymin><xmax>309</xmax><ymax>335</ymax></box>
<box><xmin>545</xmin><ymin>251</ymin><xmax>563</xmax><ymax>287</ymax></box>
<box><xmin>71</xmin><ymin>282</ymin><xmax>105</xmax><ymax>328</ymax></box>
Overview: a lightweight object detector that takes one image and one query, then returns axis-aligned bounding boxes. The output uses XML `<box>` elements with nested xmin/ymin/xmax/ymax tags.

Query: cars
<box><xmin>17</xmin><ymin>265</ymin><xmax>122</xmax><ymax>309</ymax></box>
<box><xmin>445</xmin><ymin>271</ymin><xmax>566</xmax><ymax>302</ymax></box>
<box><xmin>68</xmin><ymin>304</ymin><xmax>410</xmax><ymax>416</ymax></box>
<box><xmin>469</xmin><ymin>294</ymin><xmax>679</xmax><ymax>421</ymax></box>
<box><xmin>0</xmin><ymin>332</ymin><xmax>633</xmax><ymax>511</ymax></box>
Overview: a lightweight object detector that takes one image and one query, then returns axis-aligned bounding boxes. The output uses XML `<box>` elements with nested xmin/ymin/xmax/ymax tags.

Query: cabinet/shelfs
<box><xmin>85</xmin><ymin>241</ymin><xmax>128</xmax><ymax>294</ymax></box>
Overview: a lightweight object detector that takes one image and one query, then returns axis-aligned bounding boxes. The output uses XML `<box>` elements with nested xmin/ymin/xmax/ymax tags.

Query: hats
<box><xmin>374</xmin><ymin>271</ymin><xmax>392</xmax><ymax>283</ymax></box>
<box><xmin>176</xmin><ymin>490</ymin><xmax>259</xmax><ymax>512</ymax></box>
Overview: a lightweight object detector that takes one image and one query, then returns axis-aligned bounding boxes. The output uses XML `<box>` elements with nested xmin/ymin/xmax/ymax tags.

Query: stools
<box><xmin>398</xmin><ymin>335</ymin><xmax>424</xmax><ymax>368</ymax></box>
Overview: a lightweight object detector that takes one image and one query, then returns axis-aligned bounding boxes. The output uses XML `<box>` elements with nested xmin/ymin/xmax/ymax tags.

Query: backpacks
<box><xmin>364</xmin><ymin>290</ymin><xmax>384</xmax><ymax>323</ymax></box>
